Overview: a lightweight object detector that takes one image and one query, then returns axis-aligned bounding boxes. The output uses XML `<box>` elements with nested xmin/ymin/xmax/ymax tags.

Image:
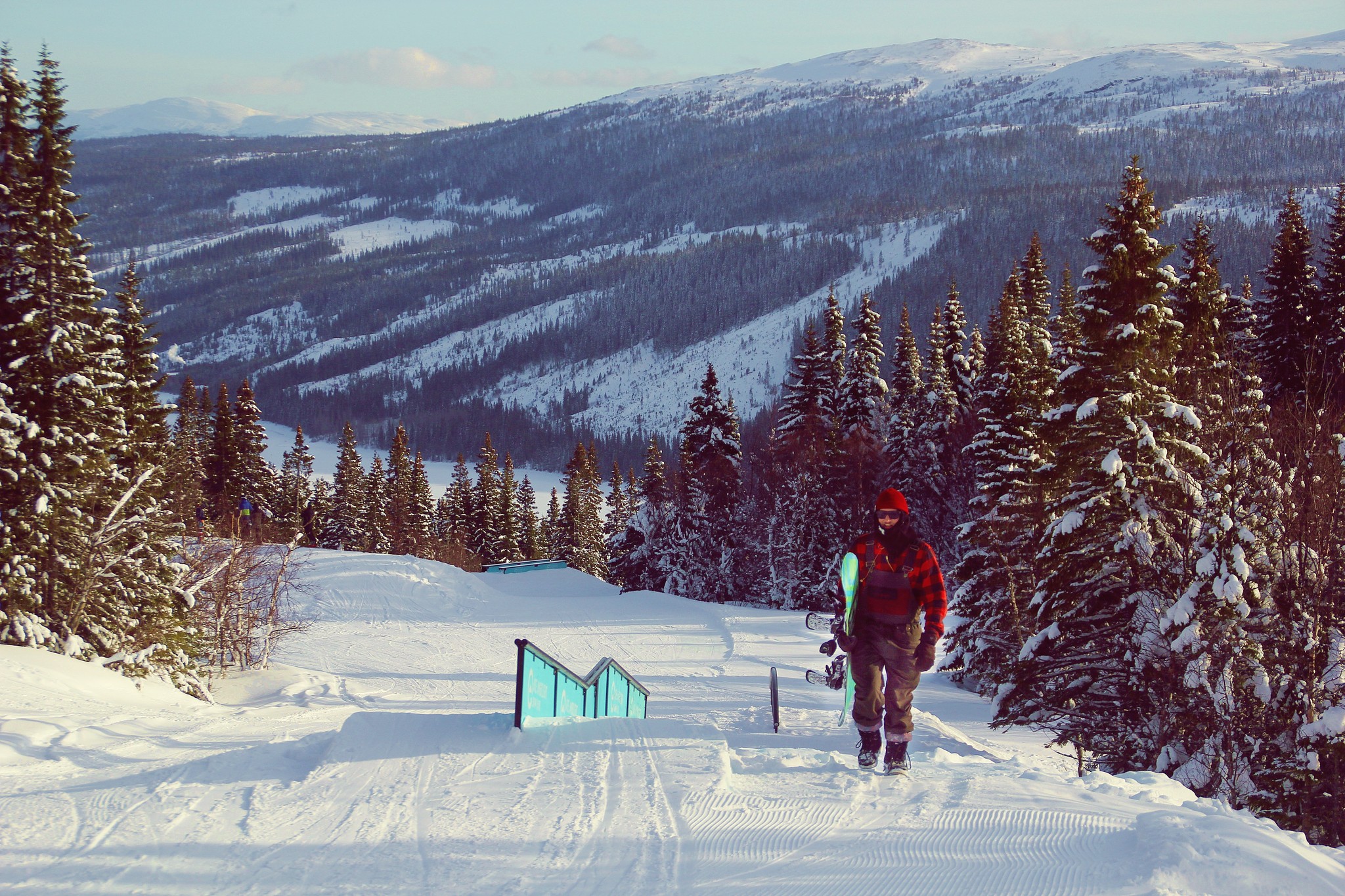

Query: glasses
<box><xmin>876</xmin><ymin>510</ymin><xmax>900</xmax><ymax>519</ymax></box>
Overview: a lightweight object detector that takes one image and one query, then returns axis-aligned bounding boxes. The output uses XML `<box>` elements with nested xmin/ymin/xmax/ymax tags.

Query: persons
<box><xmin>196</xmin><ymin>502</ymin><xmax>208</xmax><ymax>544</ymax></box>
<box><xmin>250</xmin><ymin>501</ymin><xmax>263</xmax><ymax>545</ymax></box>
<box><xmin>235</xmin><ymin>496</ymin><xmax>254</xmax><ymax>543</ymax></box>
<box><xmin>834</xmin><ymin>488</ymin><xmax>946</xmax><ymax>776</ymax></box>
<box><xmin>301</xmin><ymin>502</ymin><xmax>317</xmax><ymax>548</ymax></box>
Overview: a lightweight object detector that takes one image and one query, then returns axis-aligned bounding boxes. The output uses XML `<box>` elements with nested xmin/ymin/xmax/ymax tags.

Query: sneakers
<box><xmin>855</xmin><ymin>727</ymin><xmax>883</xmax><ymax>769</ymax></box>
<box><xmin>884</xmin><ymin>740</ymin><xmax>911</xmax><ymax>776</ymax></box>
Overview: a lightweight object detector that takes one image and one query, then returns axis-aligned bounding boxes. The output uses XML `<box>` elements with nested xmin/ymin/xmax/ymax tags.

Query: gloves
<box><xmin>837</xmin><ymin>631</ymin><xmax>857</xmax><ymax>652</ymax></box>
<box><xmin>912</xmin><ymin>632</ymin><xmax>938</xmax><ymax>672</ymax></box>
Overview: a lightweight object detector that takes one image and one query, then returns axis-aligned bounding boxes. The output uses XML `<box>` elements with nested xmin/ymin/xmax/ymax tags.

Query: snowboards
<box><xmin>836</xmin><ymin>551</ymin><xmax>859</xmax><ymax>729</ymax></box>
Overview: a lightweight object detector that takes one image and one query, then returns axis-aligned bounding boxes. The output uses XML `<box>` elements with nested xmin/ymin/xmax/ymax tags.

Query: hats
<box><xmin>876</xmin><ymin>488</ymin><xmax>909</xmax><ymax>515</ymax></box>
